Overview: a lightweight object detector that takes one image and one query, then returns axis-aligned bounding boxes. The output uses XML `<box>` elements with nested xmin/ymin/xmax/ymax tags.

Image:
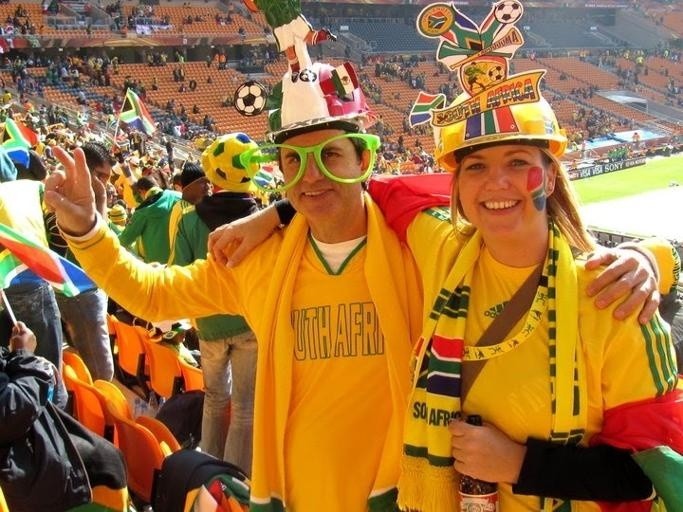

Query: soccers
<box><xmin>233</xmin><ymin>81</ymin><xmax>268</xmax><ymax>118</ymax></box>
<box><xmin>494</xmin><ymin>0</ymin><xmax>524</xmax><ymax>24</ymax></box>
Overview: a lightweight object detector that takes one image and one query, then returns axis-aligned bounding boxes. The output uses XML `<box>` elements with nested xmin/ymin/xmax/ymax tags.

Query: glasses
<box><xmin>239</xmin><ymin>132</ymin><xmax>381</xmax><ymax>193</ymax></box>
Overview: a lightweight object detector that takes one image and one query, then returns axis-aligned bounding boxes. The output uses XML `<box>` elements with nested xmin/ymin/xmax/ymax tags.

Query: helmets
<box><xmin>201</xmin><ymin>133</ymin><xmax>261</xmax><ymax>193</ymax></box>
<box><xmin>408</xmin><ymin>1</ymin><xmax>567</xmax><ymax>171</ymax></box>
<box><xmin>233</xmin><ymin>1</ymin><xmax>370</xmax><ymax>142</ymax></box>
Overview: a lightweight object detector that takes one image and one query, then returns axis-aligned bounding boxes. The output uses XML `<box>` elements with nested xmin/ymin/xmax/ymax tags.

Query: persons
<box><xmin>176</xmin><ymin>133</ymin><xmax>260</xmax><ymax>479</ymax></box>
<box><xmin>207</xmin><ymin>43</ymin><xmax>228</xmax><ymax>70</ymax></box>
<box><xmin>266</xmin><ymin>177</ymin><xmax>284</xmax><ymax>200</ymax></box>
<box><xmin>44</xmin><ymin>54</ymin><xmax>119</xmax><ymax>87</ymax></box>
<box><xmin>154</xmin><ymin>99</ymin><xmax>213</xmax><ymax>143</ymax></box>
<box><xmin>123</xmin><ymin>73</ymin><xmax>159</xmax><ymax>101</ymax></box>
<box><xmin>110</xmin><ymin>204</ymin><xmax>138</xmax><ymax>256</ymax></box>
<box><xmin>541</xmin><ymin>40</ymin><xmax>682</xmax><ymax>168</ymax></box>
<box><xmin>148</xmin><ymin>49</ymin><xmax>185</xmax><ymax>66</ymax></box>
<box><xmin>174</xmin><ymin>66</ymin><xmax>213</xmax><ymax>93</ymax></box>
<box><xmin>509</xmin><ymin>48</ymin><xmax>540</xmax><ymax>75</ymax></box>
<box><xmin>44</xmin><ymin>143</ymin><xmax>118</xmax><ymax>383</ymax></box>
<box><xmin>43</xmin><ymin>16</ymin><xmax>682</xmax><ymax>512</ymax></box>
<box><xmin>222</xmin><ymin>94</ymin><xmax>234</xmax><ymax>106</ymax></box>
<box><xmin>0</xmin><ymin>181</ymin><xmax>69</xmax><ymax>410</ymax></box>
<box><xmin>49</xmin><ymin>107</ymin><xmax>112</xmax><ymax>146</ymax></box>
<box><xmin>117</xmin><ymin>130</ymin><xmax>201</xmax><ymax>179</ymax></box>
<box><xmin>75</xmin><ymin>92</ymin><xmax>121</xmax><ymax>117</ymax></box>
<box><xmin>241</xmin><ymin>24</ymin><xmax>277</xmax><ymax>72</ymax></box>
<box><xmin>343</xmin><ymin>46</ymin><xmax>462</xmax><ymax>107</ymax></box>
<box><xmin>84</xmin><ymin>0</ymin><xmax>169</xmax><ymax>36</ymax></box>
<box><xmin>117</xmin><ymin>177</ymin><xmax>183</xmax><ymax>276</ymax></box>
<box><xmin>366</xmin><ymin>114</ymin><xmax>446</xmax><ymax>175</ymax></box>
<box><xmin>216</xmin><ymin>14</ymin><xmax>245</xmax><ymax>36</ymax></box>
<box><xmin>208</xmin><ymin>0</ymin><xmax>683</xmax><ymax>510</ymax></box>
<box><xmin>177</xmin><ymin>2</ymin><xmax>208</xmax><ymax>24</ymax></box>
<box><xmin>169</xmin><ymin>162</ymin><xmax>212</xmax><ymax>264</ymax></box>
<box><xmin>0</xmin><ymin>5</ymin><xmax>44</xmax><ymax>154</ymax></box>
<box><xmin>0</xmin><ymin>320</ymin><xmax>135</xmax><ymax>512</ymax></box>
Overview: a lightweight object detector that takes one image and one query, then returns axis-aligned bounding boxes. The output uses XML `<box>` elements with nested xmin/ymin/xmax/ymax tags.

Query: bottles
<box><xmin>457</xmin><ymin>413</ymin><xmax>500</xmax><ymax>512</ymax></box>
<box><xmin>132</xmin><ymin>390</ymin><xmax>168</xmax><ymax>419</ymax></box>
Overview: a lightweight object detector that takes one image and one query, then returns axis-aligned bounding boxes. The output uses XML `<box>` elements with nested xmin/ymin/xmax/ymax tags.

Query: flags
<box><xmin>0</xmin><ymin>224</ymin><xmax>97</xmax><ymax>335</ymax></box>
<box><xmin>0</xmin><ymin>26</ymin><xmax>11</xmax><ymax>54</ymax></box>
<box><xmin>113</xmin><ymin>88</ymin><xmax>158</xmax><ymax>137</ymax></box>
